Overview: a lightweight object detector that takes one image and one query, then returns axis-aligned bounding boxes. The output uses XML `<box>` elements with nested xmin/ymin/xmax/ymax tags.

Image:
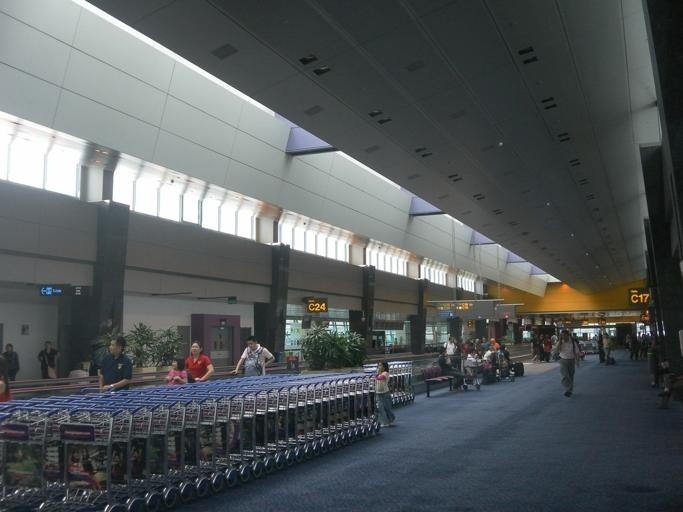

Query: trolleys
<box><xmin>450</xmin><ymin>352</ymin><xmax>480</xmax><ymax>390</ymax></box>
<box><xmin>494</xmin><ymin>350</ymin><xmax>516</xmax><ymax>382</ymax></box>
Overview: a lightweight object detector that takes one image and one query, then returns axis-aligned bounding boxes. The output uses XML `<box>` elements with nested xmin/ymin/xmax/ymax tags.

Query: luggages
<box><xmin>512</xmin><ymin>361</ymin><xmax>524</xmax><ymax>377</ymax></box>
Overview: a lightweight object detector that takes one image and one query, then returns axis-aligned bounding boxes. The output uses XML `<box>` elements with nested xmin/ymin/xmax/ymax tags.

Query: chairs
<box><xmin>421</xmin><ymin>366</ymin><xmax>454</xmax><ymax>399</ymax></box>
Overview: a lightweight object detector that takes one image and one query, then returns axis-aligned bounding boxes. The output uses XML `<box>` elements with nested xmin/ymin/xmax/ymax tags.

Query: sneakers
<box><xmin>379</xmin><ymin>415</ymin><xmax>399</xmax><ymax>427</ymax></box>
<box><xmin>564</xmin><ymin>391</ymin><xmax>573</xmax><ymax>397</ymax></box>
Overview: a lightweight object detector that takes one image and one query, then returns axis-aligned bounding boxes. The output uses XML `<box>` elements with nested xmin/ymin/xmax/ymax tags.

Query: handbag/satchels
<box><xmin>48</xmin><ymin>367</ymin><xmax>57</xmax><ymax>379</ymax></box>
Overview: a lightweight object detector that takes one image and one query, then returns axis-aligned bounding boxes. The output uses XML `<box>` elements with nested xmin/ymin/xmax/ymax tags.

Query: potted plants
<box><xmin>125</xmin><ymin>322</ymin><xmax>187</xmax><ymax>382</ymax></box>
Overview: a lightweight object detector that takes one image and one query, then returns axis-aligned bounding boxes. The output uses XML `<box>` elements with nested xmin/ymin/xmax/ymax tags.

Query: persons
<box><xmin>556</xmin><ymin>329</ymin><xmax>580</xmax><ymax>396</ymax></box>
<box><xmin>370</xmin><ymin>360</ymin><xmax>395</xmax><ymax>427</ymax></box>
<box><xmin>625</xmin><ymin>330</ymin><xmax>683</xmax><ymax>409</ymax></box>
<box><xmin>1</xmin><ymin>344</ymin><xmax>20</xmax><ymax>381</ymax></box>
<box><xmin>0</xmin><ymin>357</ymin><xmax>11</xmax><ymax>401</ymax></box>
<box><xmin>393</xmin><ymin>338</ymin><xmax>401</xmax><ymax>353</ymax></box>
<box><xmin>591</xmin><ymin>332</ymin><xmax>617</xmax><ymax>366</ymax></box>
<box><xmin>38</xmin><ymin>341</ymin><xmax>59</xmax><ymax>379</ymax></box>
<box><xmin>231</xmin><ymin>336</ymin><xmax>275</xmax><ymax>376</ymax></box>
<box><xmin>185</xmin><ymin>340</ymin><xmax>215</xmax><ymax>383</ymax></box>
<box><xmin>437</xmin><ymin>336</ymin><xmax>513</xmax><ymax>391</ymax></box>
<box><xmin>165</xmin><ymin>358</ymin><xmax>189</xmax><ymax>385</ymax></box>
<box><xmin>97</xmin><ymin>336</ymin><xmax>133</xmax><ymax>392</ymax></box>
<box><xmin>529</xmin><ymin>328</ymin><xmax>585</xmax><ymax>366</ymax></box>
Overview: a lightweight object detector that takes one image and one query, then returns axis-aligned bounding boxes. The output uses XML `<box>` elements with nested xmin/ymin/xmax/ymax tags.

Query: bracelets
<box><xmin>110</xmin><ymin>383</ymin><xmax>115</xmax><ymax>388</ymax></box>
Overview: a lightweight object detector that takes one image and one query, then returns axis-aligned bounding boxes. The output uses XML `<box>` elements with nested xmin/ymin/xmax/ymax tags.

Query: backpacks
<box><xmin>242</xmin><ymin>346</ymin><xmax>264</xmax><ymax>377</ymax></box>
